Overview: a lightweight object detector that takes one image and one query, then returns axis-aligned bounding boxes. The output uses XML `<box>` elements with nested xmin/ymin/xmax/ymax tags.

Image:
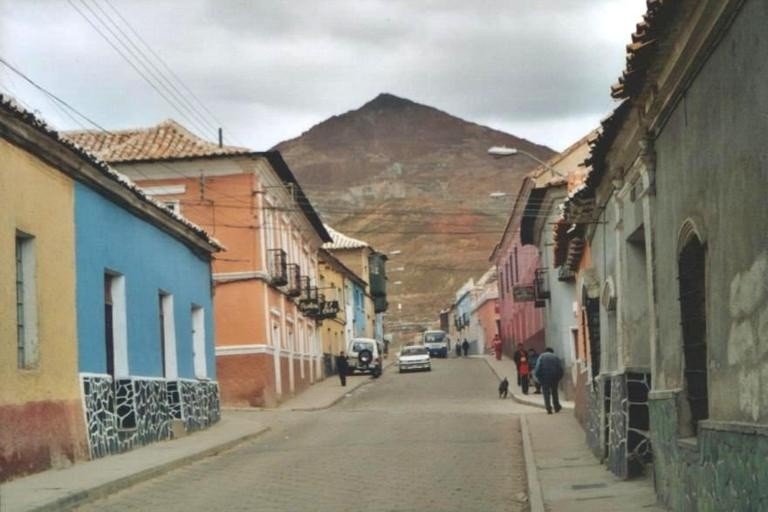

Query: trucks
<box><xmin>423</xmin><ymin>330</ymin><xmax>447</xmax><ymax>357</ymax></box>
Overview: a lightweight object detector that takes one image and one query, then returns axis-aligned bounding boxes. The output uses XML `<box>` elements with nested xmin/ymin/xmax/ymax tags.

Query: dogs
<box><xmin>498</xmin><ymin>377</ymin><xmax>509</xmax><ymax>398</ymax></box>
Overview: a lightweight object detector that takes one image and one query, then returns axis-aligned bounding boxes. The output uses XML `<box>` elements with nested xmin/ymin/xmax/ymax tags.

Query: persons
<box><xmin>462</xmin><ymin>338</ymin><xmax>470</xmax><ymax>355</ymax></box>
<box><xmin>494</xmin><ymin>336</ymin><xmax>502</xmax><ymax>360</ymax></box>
<box><xmin>534</xmin><ymin>348</ymin><xmax>564</xmax><ymax>415</ymax></box>
<box><xmin>337</xmin><ymin>351</ymin><xmax>348</xmax><ymax>386</ymax></box>
<box><xmin>519</xmin><ymin>356</ymin><xmax>530</xmax><ymax>394</ymax></box>
<box><xmin>513</xmin><ymin>343</ymin><xmax>528</xmax><ymax>385</ymax></box>
<box><xmin>528</xmin><ymin>348</ymin><xmax>541</xmax><ymax>394</ymax></box>
<box><xmin>456</xmin><ymin>339</ymin><xmax>461</xmax><ymax>356</ymax></box>
<box><xmin>491</xmin><ymin>334</ymin><xmax>497</xmax><ymax>348</ymax></box>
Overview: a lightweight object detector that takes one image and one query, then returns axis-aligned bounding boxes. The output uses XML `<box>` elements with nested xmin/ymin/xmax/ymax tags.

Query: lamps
<box><xmin>488</xmin><ymin>146</ymin><xmax>576</xmax><ymax>188</ymax></box>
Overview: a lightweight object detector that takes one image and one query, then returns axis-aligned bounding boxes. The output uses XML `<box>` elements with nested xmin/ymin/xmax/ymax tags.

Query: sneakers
<box><xmin>547</xmin><ymin>409</ymin><xmax>552</xmax><ymax>413</ymax></box>
<box><xmin>555</xmin><ymin>404</ymin><xmax>561</xmax><ymax>412</ymax></box>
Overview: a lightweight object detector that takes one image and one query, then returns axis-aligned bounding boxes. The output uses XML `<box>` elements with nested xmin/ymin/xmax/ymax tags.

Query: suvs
<box><xmin>346</xmin><ymin>337</ymin><xmax>381</xmax><ymax>377</ymax></box>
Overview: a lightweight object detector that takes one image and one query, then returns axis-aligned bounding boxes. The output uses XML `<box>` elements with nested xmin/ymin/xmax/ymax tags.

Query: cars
<box><xmin>398</xmin><ymin>345</ymin><xmax>431</xmax><ymax>373</ymax></box>
